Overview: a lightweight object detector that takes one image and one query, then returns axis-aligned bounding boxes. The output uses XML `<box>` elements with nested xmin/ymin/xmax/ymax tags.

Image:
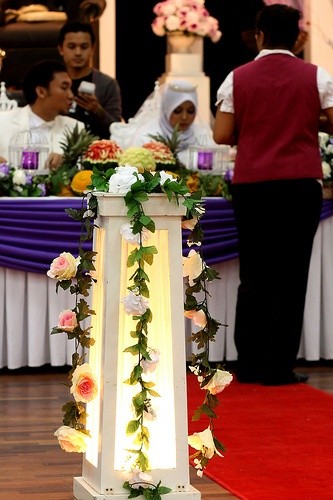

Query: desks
<box><xmin>0</xmin><ymin>194</ymin><xmax>333</xmax><ymax>369</ymax></box>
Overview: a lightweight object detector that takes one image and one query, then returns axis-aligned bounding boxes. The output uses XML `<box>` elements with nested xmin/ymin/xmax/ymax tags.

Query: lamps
<box><xmin>72</xmin><ymin>190</ymin><xmax>202</xmax><ymax>500</ymax></box>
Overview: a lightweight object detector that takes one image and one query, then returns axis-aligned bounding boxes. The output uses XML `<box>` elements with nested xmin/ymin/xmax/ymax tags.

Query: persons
<box><xmin>0</xmin><ymin>62</ymin><xmax>88</xmax><ymax>176</ymax></box>
<box><xmin>56</xmin><ymin>20</ymin><xmax>123</xmax><ymax>140</ymax></box>
<box><xmin>212</xmin><ymin>5</ymin><xmax>333</xmax><ymax>386</ymax></box>
<box><xmin>140</xmin><ymin>76</ymin><xmax>216</xmax><ymax>167</ymax></box>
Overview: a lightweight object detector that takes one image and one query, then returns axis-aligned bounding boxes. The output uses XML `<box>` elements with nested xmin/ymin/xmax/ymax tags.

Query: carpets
<box><xmin>186</xmin><ymin>368</ymin><xmax>333</xmax><ymax>500</ymax></box>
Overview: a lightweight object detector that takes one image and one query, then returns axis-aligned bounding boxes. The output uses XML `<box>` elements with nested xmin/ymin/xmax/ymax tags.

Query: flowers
<box><xmin>0</xmin><ymin>162</ymin><xmax>46</xmax><ymax>197</ymax></box>
<box><xmin>47</xmin><ymin>120</ymin><xmax>234</xmax><ymax>500</ymax></box>
<box><xmin>318</xmin><ymin>131</ymin><xmax>333</xmax><ymax>186</ymax></box>
<box><xmin>151</xmin><ymin>0</ymin><xmax>223</xmax><ymax>41</ymax></box>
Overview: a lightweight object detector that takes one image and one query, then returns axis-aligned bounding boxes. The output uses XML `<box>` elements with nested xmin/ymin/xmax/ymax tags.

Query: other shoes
<box><xmin>239</xmin><ymin>374</ymin><xmax>259</xmax><ymax>383</ymax></box>
<box><xmin>284</xmin><ymin>372</ymin><xmax>309</xmax><ymax>382</ymax></box>
